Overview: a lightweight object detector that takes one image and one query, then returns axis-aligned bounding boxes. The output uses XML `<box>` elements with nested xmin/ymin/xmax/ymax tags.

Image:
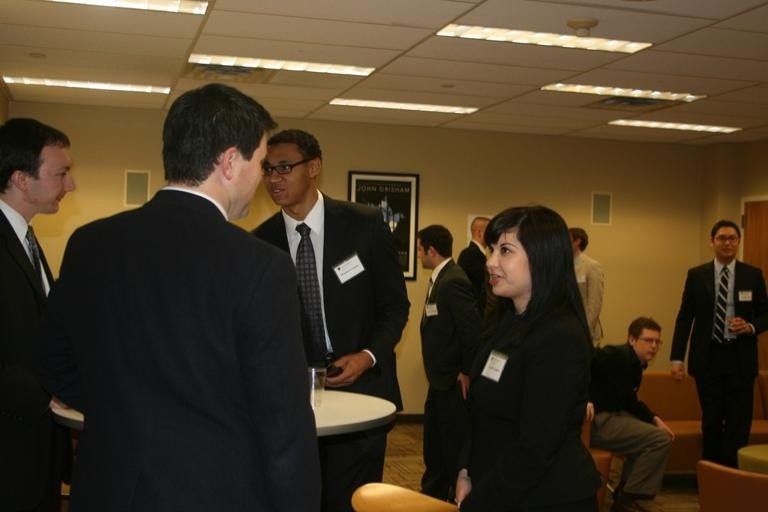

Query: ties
<box><xmin>712</xmin><ymin>267</ymin><xmax>729</xmax><ymax>346</ymax></box>
<box><xmin>26</xmin><ymin>225</ymin><xmax>42</xmax><ymax>279</ymax></box>
<box><xmin>296</xmin><ymin>223</ymin><xmax>327</xmax><ymax>367</ymax></box>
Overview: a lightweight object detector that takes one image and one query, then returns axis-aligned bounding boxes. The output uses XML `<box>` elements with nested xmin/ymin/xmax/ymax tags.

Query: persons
<box><xmin>42</xmin><ymin>83</ymin><xmax>324</xmax><ymax>510</ymax></box>
<box><xmin>246</xmin><ymin>127</ymin><xmax>411</xmax><ymax>510</ymax></box>
<box><xmin>586</xmin><ymin>317</ymin><xmax>675</xmax><ymax>511</ymax></box>
<box><xmin>0</xmin><ymin>118</ymin><xmax>77</xmax><ymax>510</ymax></box>
<box><xmin>670</xmin><ymin>220</ymin><xmax>767</xmax><ymax>469</ymax></box>
<box><xmin>417</xmin><ymin>206</ymin><xmax>606</xmax><ymax>511</ymax></box>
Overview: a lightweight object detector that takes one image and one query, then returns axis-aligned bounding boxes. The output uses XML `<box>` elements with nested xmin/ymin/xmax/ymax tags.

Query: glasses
<box><xmin>264</xmin><ymin>159</ymin><xmax>312</xmax><ymax>177</ymax></box>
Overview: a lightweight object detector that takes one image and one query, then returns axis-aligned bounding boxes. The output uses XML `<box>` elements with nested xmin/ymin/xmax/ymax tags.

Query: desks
<box><xmin>50</xmin><ymin>388</ymin><xmax>397</xmax><ymax>437</ymax></box>
<box><xmin>738</xmin><ymin>443</ymin><xmax>767</xmax><ymax>473</ymax></box>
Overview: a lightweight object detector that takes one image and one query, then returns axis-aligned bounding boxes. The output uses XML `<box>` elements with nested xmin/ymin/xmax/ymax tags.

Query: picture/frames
<box><xmin>346</xmin><ymin>169</ymin><xmax>419</xmax><ymax>283</ymax></box>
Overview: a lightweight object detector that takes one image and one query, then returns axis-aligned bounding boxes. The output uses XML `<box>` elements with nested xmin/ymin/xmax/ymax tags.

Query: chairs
<box><xmin>695</xmin><ymin>458</ymin><xmax>768</xmax><ymax>512</ymax></box>
<box><xmin>580</xmin><ymin>411</ymin><xmax>612</xmax><ymax>512</ymax></box>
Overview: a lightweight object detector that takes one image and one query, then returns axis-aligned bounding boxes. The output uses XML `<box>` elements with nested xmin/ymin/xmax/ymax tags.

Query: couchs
<box><xmin>634</xmin><ymin>369</ymin><xmax>768</xmax><ymax>470</ymax></box>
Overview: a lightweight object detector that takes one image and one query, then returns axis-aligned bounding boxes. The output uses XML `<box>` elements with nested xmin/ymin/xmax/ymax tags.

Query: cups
<box><xmin>308</xmin><ymin>366</ymin><xmax>329</xmax><ymax>414</ymax></box>
<box><xmin>723</xmin><ymin>314</ymin><xmax>739</xmax><ymax>338</ymax></box>
